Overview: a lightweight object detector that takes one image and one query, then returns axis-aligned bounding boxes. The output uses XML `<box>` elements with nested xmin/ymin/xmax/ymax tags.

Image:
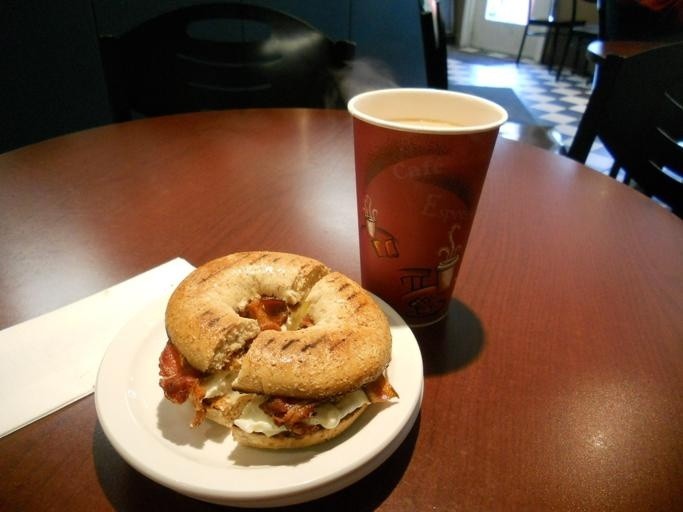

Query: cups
<box><xmin>347</xmin><ymin>87</ymin><xmax>510</xmax><ymax>329</ymax></box>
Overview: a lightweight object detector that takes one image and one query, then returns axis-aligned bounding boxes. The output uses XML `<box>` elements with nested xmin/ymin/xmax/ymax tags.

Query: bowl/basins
<box><xmin>91</xmin><ymin>285</ymin><xmax>426</xmax><ymax>511</ymax></box>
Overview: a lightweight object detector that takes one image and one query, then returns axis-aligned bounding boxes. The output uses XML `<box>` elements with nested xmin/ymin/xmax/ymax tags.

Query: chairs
<box><xmin>514</xmin><ymin>1</ymin><xmax>583</xmax><ymax>72</ymax></box>
<box><xmin>559</xmin><ymin>1</ymin><xmax>681</xmax><ymax>218</ymax></box>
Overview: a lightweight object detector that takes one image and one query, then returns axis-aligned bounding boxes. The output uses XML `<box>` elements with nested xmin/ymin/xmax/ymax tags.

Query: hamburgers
<box><xmin>159</xmin><ymin>251</ymin><xmax>399</xmax><ymax>449</ymax></box>
